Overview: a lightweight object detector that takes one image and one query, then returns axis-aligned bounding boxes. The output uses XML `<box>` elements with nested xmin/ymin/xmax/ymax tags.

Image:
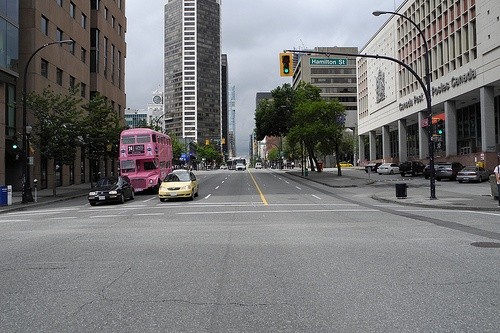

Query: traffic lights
<box><xmin>280</xmin><ymin>53</ymin><xmax>293</xmax><ymax>76</ymax></box>
<box><xmin>11</xmin><ymin>144</ymin><xmax>17</xmax><ymax>150</ymax></box>
<box><xmin>435</xmin><ymin>128</ymin><xmax>443</xmax><ymax>136</ymax></box>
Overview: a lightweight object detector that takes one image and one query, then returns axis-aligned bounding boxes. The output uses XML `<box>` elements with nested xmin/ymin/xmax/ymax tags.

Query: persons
<box><xmin>291</xmin><ymin>160</ymin><xmax>294</xmax><ymax>169</ymax></box>
<box><xmin>493</xmin><ymin>157</ymin><xmax>500</xmax><ymax>206</ymax></box>
<box><xmin>281</xmin><ymin>162</ymin><xmax>284</xmax><ymax>169</ymax></box>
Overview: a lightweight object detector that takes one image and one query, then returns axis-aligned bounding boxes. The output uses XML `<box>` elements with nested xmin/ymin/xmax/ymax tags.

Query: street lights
<box><xmin>25</xmin><ymin>124</ymin><xmax>34</xmax><ymax>202</ymax></box>
<box><xmin>21</xmin><ymin>38</ymin><xmax>75</xmax><ymax>205</ymax></box>
<box><xmin>371</xmin><ymin>10</ymin><xmax>438</xmax><ymax>200</ymax></box>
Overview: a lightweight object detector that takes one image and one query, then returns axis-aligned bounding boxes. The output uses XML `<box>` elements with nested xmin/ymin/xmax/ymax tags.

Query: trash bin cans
<box><xmin>0</xmin><ymin>185</ymin><xmax>8</xmax><ymax>206</ymax></box>
<box><xmin>395</xmin><ymin>184</ymin><xmax>407</xmax><ymax>199</ymax></box>
<box><xmin>489</xmin><ymin>174</ymin><xmax>499</xmax><ymax>196</ymax></box>
<box><xmin>318</xmin><ymin>161</ymin><xmax>323</xmax><ymax>171</ymax></box>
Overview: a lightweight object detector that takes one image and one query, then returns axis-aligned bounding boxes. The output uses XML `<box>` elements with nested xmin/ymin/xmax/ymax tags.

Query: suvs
<box><xmin>398</xmin><ymin>160</ymin><xmax>425</xmax><ymax>177</ymax></box>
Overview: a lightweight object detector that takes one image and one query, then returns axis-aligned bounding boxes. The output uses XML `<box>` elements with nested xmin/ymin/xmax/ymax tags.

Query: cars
<box><xmin>376</xmin><ymin>162</ymin><xmax>400</xmax><ymax>176</ymax></box>
<box><xmin>336</xmin><ymin>162</ymin><xmax>353</xmax><ymax>168</ymax></box>
<box><xmin>436</xmin><ymin>161</ymin><xmax>466</xmax><ymax>182</ymax></box>
<box><xmin>364</xmin><ymin>162</ymin><xmax>382</xmax><ymax>173</ymax></box>
<box><xmin>159</xmin><ymin>171</ymin><xmax>199</xmax><ymax>202</ymax></box>
<box><xmin>423</xmin><ymin>162</ymin><xmax>437</xmax><ymax>179</ymax></box>
<box><xmin>235</xmin><ymin>163</ymin><xmax>246</xmax><ymax>171</ymax></box>
<box><xmin>87</xmin><ymin>175</ymin><xmax>135</xmax><ymax>206</ymax></box>
<box><xmin>456</xmin><ymin>165</ymin><xmax>490</xmax><ymax>184</ymax></box>
<box><xmin>254</xmin><ymin>163</ymin><xmax>262</xmax><ymax>170</ymax></box>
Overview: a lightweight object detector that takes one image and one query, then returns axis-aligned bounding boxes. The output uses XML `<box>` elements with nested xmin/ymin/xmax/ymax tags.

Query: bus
<box><xmin>119</xmin><ymin>128</ymin><xmax>172</xmax><ymax>193</ymax></box>
<box><xmin>226</xmin><ymin>158</ymin><xmax>247</xmax><ymax>170</ymax></box>
